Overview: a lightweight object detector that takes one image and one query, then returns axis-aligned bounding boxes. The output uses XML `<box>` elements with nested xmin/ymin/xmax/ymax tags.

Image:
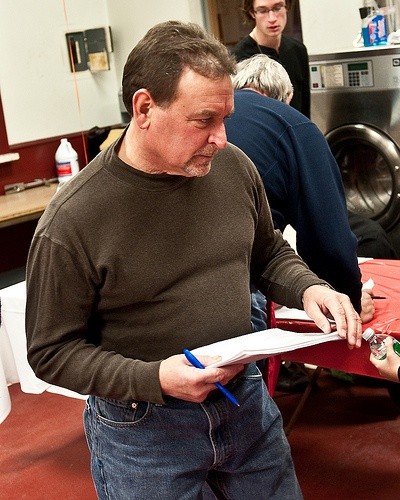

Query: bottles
<box><xmin>361</xmin><ymin>327</ymin><xmax>400</xmax><ymax>360</ymax></box>
<box><xmin>55</xmin><ymin>138</ymin><xmax>80</xmax><ymax>184</ymax></box>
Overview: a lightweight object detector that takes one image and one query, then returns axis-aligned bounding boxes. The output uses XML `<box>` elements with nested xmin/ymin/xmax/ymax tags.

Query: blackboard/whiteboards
<box><xmin>0</xmin><ymin>0</ymin><xmax>123</xmax><ymax>150</ymax></box>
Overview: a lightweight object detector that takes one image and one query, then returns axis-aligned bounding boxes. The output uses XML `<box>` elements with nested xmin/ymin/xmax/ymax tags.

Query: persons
<box><xmin>370</xmin><ymin>337</ymin><xmax>400</xmax><ymax>382</ymax></box>
<box><xmin>235</xmin><ymin>1</ymin><xmax>310</xmax><ymax>120</ymax></box>
<box><xmin>223</xmin><ymin>53</ymin><xmax>376</xmax><ymax>391</ymax></box>
<box><xmin>25</xmin><ymin>20</ymin><xmax>362</xmax><ymax>499</ymax></box>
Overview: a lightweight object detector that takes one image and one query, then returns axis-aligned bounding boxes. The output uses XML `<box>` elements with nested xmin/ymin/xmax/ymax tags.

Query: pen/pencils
<box><xmin>183</xmin><ymin>348</ymin><xmax>240</xmax><ymax>407</ymax></box>
<box><xmin>371</xmin><ymin>296</ymin><xmax>386</xmax><ymax>299</ymax></box>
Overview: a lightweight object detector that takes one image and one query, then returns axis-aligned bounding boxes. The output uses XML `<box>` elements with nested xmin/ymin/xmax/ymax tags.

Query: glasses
<box><xmin>251</xmin><ymin>2</ymin><xmax>287</xmax><ymax>15</ymax></box>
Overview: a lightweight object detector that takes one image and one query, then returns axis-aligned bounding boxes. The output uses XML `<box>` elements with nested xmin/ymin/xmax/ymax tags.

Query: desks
<box><xmin>267</xmin><ymin>257</ymin><xmax>400</xmax><ymax>398</ymax></box>
<box><xmin>0</xmin><ymin>179</ymin><xmax>68</xmax><ymax>230</ymax></box>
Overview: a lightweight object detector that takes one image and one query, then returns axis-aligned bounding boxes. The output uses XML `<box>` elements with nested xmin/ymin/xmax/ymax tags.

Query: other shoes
<box><xmin>275</xmin><ymin>366</ymin><xmax>307</xmax><ymax>393</ymax></box>
